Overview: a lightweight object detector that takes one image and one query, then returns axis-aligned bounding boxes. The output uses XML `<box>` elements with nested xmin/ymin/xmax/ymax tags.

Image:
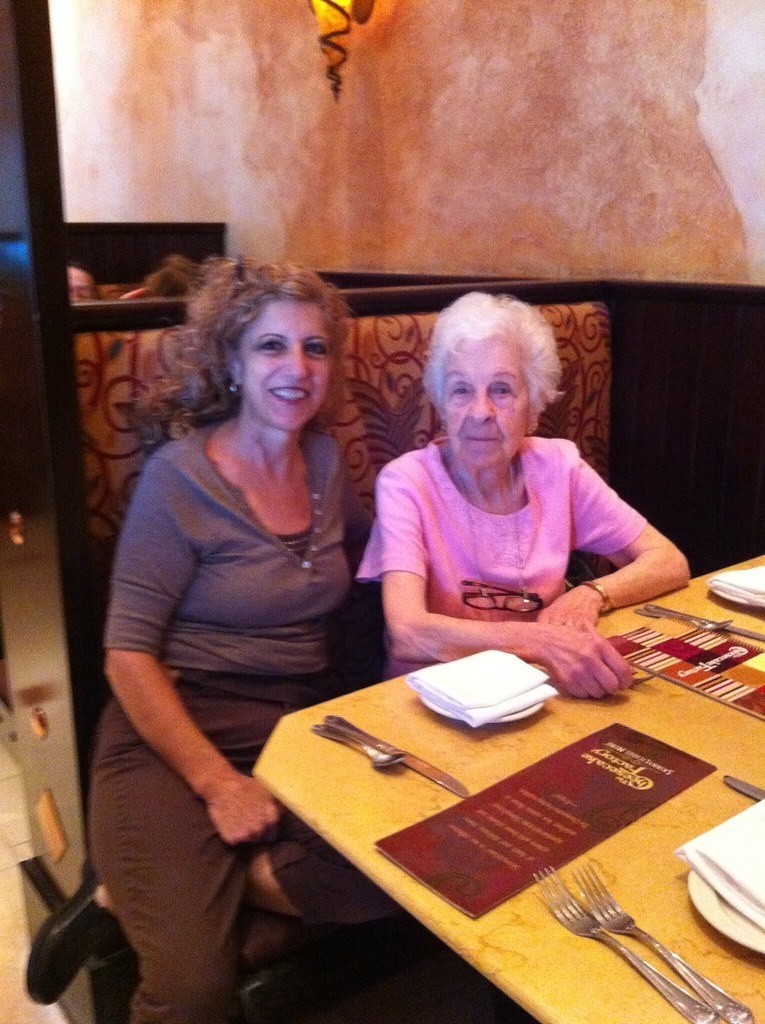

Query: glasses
<box><xmin>458</xmin><ymin>577</ymin><xmax>542</xmax><ymax>613</ymax></box>
<box><xmin>64</xmin><ymin>284</ymin><xmax>97</xmax><ymax>300</ymax></box>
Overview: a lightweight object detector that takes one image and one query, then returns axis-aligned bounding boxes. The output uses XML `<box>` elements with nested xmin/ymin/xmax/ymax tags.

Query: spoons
<box><xmin>311</xmin><ymin>724</ymin><xmax>406</xmax><ymax>766</ymax></box>
<box><xmin>632</xmin><ymin>609</ymin><xmax>733</xmax><ymax>630</ymax></box>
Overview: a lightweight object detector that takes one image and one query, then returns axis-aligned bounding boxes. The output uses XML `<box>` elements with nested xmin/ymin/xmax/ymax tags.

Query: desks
<box><xmin>251</xmin><ymin>556</ymin><xmax>764</xmax><ymax>1024</ymax></box>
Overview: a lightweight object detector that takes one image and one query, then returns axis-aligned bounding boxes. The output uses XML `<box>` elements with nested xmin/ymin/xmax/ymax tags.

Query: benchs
<box><xmin>71</xmin><ymin>278</ymin><xmax>618</xmax><ymax>1024</ymax></box>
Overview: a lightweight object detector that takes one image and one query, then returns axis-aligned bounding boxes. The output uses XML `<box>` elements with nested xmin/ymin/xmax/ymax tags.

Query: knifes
<box><xmin>325</xmin><ymin>715</ymin><xmax>469</xmax><ymax>798</ymax></box>
<box><xmin>644</xmin><ymin>604</ymin><xmax>765</xmax><ymax>641</ymax></box>
<box><xmin>722</xmin><ymin>775</ymin><xmax>765</xmax><ymax>801</ymax></box>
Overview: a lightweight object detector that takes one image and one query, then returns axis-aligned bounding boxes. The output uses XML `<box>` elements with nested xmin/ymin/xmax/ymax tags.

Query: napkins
<box><xmin>406</xmin><ymin>650</ymin><xmax>559</xmax><ymax>728</ymax></box>
<box><xmin>675</xmin><ymin>800</ymin><xmax>763</xmax><ymax>932</ymax></box>
<box><xmin>707</xmin><ymin>566</ymin><xmax>765</xmax><ymax>608</ymax></box>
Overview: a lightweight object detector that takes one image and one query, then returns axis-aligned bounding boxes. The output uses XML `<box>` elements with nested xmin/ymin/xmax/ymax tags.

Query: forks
<box><xmin>569</xmin><ymin>863</ymin><xmax>754</xmax><ymax>1024</ymax></box>
<box><xmin>533</xmin><ymin>866</ymin><xmax>720</xmax><ymax>1024</ymax></box>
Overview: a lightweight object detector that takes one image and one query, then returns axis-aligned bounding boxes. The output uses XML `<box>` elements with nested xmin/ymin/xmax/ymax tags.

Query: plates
<box><xmin>687</xmin><ymin>869</ymin><xmax>765</xmax><ymax>955</ymax></box>
<box><xmin>419</xmin><ymin>693</ymin><xmax>544</xmax><ymax>723</ymax></box>
<box><xmin>710</xmin><ymin>588</ymin><xmax>765</xmax><ymax>607</ymax></box>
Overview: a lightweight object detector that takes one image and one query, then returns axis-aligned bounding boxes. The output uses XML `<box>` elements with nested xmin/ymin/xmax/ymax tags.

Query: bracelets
<box><xmin>579</xmin><ymin>579</ymin><xmax>611</xmax><ymax>613</ymax></box>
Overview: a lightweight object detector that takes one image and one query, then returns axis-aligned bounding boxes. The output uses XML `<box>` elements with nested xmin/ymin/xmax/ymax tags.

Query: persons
<box><xmin>372</xmin><ymin>287</ymin><xmax>692</xmax><ymax>693</ymax></box>
<box><xmin>88</xmin><ymin>251</ymin><xmax>373</xmax><ymax>1023</ymax></box>
<box><xmin>66</xmin><ymin>254</ymin><xmax>201</xmax><ymax>302</ymax></box>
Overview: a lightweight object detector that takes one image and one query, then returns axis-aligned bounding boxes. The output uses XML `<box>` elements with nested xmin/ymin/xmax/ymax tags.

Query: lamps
<box><xmin>306</xmin><ymin>0</ymin><xmax>374</xmax><ymax>103</ymax></box>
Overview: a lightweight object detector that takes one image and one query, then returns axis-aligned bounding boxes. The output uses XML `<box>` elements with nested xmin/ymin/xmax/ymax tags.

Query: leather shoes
<box><xmin>23</xmin><ymin>872</ymin><xmax>118</xmax><ymax>1007</ymax></box>
